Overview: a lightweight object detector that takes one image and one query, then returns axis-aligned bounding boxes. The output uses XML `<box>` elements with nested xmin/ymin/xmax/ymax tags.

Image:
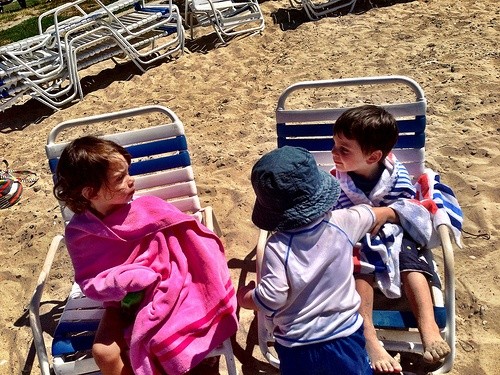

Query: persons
<box><xmin>326</xmin><ymin>105</ymin><xmax>451</xmax><ymax>375</ymax></box>
<box><xmin>236</xmin><ymin>145</ymin><xmax>404</xmax><ymax>375</ymax></box>
<box><xmin>51</xmin><ymin>135</ymin><xmax>239</xmax><ymax>375</ymax></box>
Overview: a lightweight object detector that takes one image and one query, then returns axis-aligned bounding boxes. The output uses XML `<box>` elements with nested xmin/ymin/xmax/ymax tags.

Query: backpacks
<box><xmin>0</xmin><ymin>159</ymin><xmax>24</xmax><ymax>209</ymax></box>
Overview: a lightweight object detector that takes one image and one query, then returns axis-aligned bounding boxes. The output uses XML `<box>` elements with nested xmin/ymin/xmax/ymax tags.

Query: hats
<box><xmin>251</xmin><ymin>146</ymin><xmax>342</xmax><ymax>232</ymax></box>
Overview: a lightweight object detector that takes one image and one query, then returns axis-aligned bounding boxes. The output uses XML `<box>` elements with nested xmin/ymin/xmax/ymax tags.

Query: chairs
<box><xmin>0</xmin><ymin>0</ymin><xmax>265</xmax><ymax>111</ymax></box>
<box><xmin>290</xmin><ymin>0</ymin><xmax>358</xmax><ymax>21</ymax></box>
<box><xmin>256</xmin><ymin>75</ymin><xmax>466</xmax><ymax>375</ymax></box>
<box><xmin>29</xmin><ymin>105</ymin><xmax>237</xmax><ymax>375</ymax></box>
